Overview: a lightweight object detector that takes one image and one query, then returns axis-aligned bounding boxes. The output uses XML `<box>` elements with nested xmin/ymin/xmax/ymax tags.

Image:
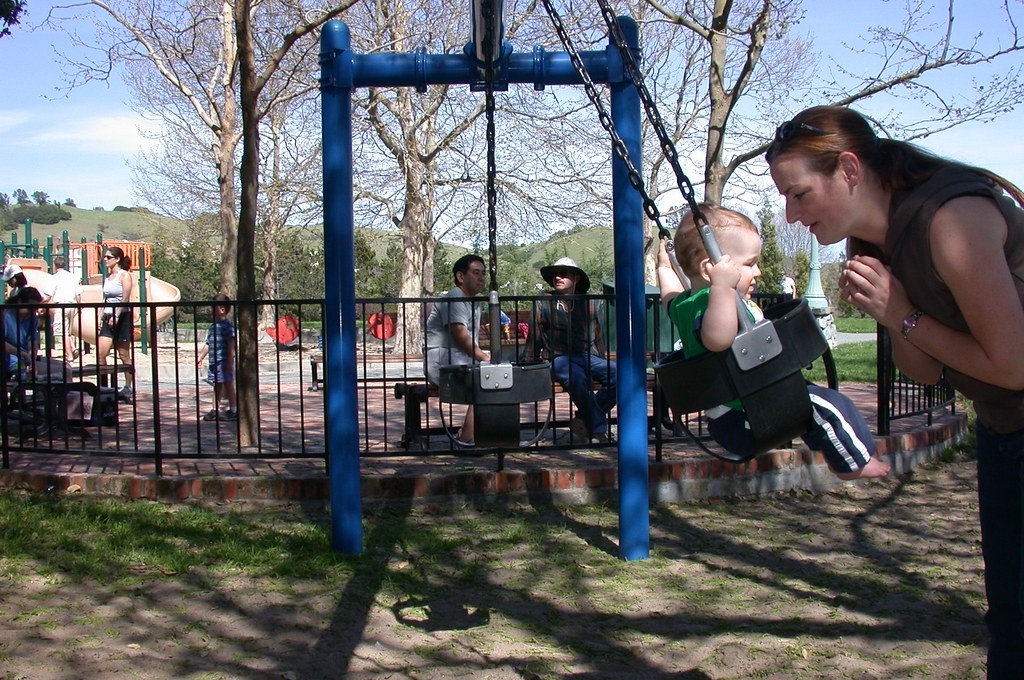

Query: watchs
<box><xmin>901</xmin><ymin>308</ymin><xmax>923</xmax><ymax>338</ymax></box>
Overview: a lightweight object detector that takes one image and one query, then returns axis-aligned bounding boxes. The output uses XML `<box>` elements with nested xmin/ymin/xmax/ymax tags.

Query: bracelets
<box><xmin>114</xmin><ymin>314</ymin><xmax>118</xmax><ymax>318</ymax></box>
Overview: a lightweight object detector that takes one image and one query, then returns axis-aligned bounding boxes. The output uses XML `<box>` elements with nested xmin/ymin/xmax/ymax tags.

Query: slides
<box><xmin>21</xmin><ymin>269</ymin><xmax>182</xmax><ymax>349</ymax></box>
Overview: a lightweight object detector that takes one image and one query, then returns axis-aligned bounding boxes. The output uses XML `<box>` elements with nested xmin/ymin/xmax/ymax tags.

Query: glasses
<box><xmin>776</xmin><ymin>120</ymin><xmax>824</xmax><ymax>143</ymax></box>
<box><xmin>104</xmin><ymin>255</ymin><xmax>117</xmax><ymax>260</ymax></box>
<box><xmin>551</xmin><ymin>270</ymin><xmax>576</xmax><ymax>279</ymax></box>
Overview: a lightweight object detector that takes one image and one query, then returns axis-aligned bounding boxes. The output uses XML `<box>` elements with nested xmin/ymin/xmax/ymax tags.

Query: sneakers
<box><xmin>219</xmin><ymin>410</ymin><xmax>238</xmax><ymax>421</ymax></box>
<box><xmin>203</xmin><ymin>409</ymin><xmax>217</xmax><ymax>421</ymax></box>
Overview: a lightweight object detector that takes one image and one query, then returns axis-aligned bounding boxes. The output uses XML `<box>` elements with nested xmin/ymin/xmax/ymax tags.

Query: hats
<box><xmin>541</xmin><ymin>257</ymin><xmax>591</xmax><ymax>294</ymax></box>
<box><xmin>1</xmin><ymin>265</ymin><xmax>23</xmax><ymax>281</ymax></box>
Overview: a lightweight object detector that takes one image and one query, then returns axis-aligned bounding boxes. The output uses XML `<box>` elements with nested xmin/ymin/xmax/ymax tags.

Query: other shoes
<box><xmin>71</xmin><ymin>349</ymin><xmax>79</xmax><ymax>360</ymax></box>
<box><xmin>568</xmin><ymin>418</ymin><xmax>616</xmax><ymax>444</ymax></box>
<box><xmin>65</xmin><ymin>355</ymin><xmax>74</xmax><ymax>362</ymax></box>
<box><xmin>119</xmin><ymin>386</ymin><xmax>135</xmax><ymax>398</ymax></box>
<box><xmin>452</xmin><ymin>431</ymin><xmax>474</xmax><ymax>449</ymax></box>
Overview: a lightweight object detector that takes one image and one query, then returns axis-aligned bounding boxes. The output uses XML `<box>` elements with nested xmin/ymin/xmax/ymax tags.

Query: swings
<box><xmin>537</xmin><ymin>1</ymin><xmax>830</xmax><ymax>468</ymax></box>
<box><xmin>435</xmin><ymin>2</ymin><xmax>558</xmax><ymax>458</ymax></box>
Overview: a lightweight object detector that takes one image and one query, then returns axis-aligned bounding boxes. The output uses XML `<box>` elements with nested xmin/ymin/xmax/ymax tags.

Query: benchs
<box><xmin>4</xmin><ymin>363</ymin><xmax>131</xmax><ymax>443</ymax></box>
<box><xmin>309</xmin><ymin>351</ymin><xmax>656</xmax><ymax>449</ymax></box>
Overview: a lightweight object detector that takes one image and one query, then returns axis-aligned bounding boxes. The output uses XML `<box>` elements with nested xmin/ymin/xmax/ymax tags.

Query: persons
<box><xmin>0</xmin><ymin>287</ymin><xmax>115</xmax><ymax>437</ymax></box>
<box><xmin>658</xmin><ymin>203</ymin><xmax>892</xmax><ymax>479</ymax></box>
<box><xmin>99</xmin><ymin>247</ymin><xmax>133</xmax><ymax>399</ymax></box>
<box><xmin>198</xmin><ymin>294</ymin><xmax>237</xmax><ymax>422</ymax></box>
<box><xmin>764</xmin><ymin>103</ymin><xmax>1024</xmax><ymax>680</ymax></box>
<box><xmin>2</xmin><ymin>264</ymin><xmax>50</xmax><ymax>360</ymax></box>
<box><xmin>423</xmin><ymin>255</ymin><xmax>489</xmax><ymax>447</ymax></box>
<box><xmin>520</xmin><ymin>257</ymin><xmax>617</xmax><ymax>445</ymax></box>
<box><xmin>42</xmin><ymin>257</ymin><xmax>84</xmax><ymax>365</ymax></box>
<box><xmin>486</xmin><ymin>307</ymin><xmax>512</xmax><ymax>341</ymax></box>
